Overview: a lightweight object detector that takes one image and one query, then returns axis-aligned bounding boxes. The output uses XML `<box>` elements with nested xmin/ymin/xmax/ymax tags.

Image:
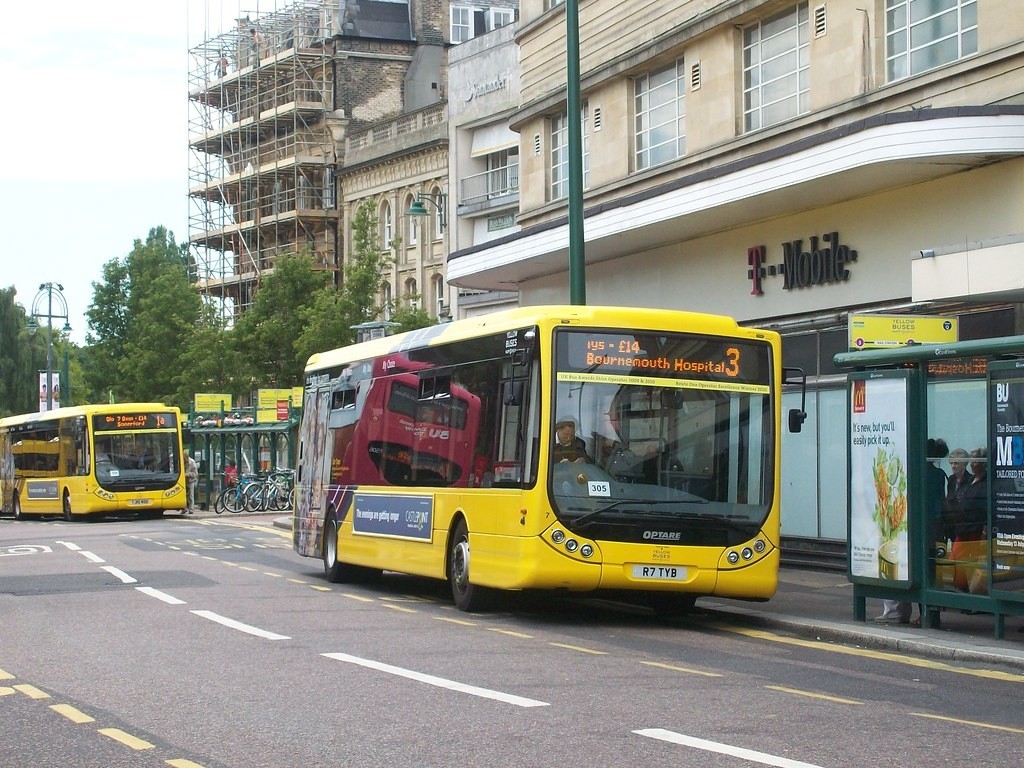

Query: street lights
<box><xmin>23</xmin><ymin>281</ymin><xmax>74</xmax><ymax>410</ymax></box>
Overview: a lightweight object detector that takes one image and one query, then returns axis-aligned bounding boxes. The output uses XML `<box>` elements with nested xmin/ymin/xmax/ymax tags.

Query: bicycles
<box><xmin>213</xmin><ymin>469</ymin><xmax>295</xmax><ymax>514</ymax></box>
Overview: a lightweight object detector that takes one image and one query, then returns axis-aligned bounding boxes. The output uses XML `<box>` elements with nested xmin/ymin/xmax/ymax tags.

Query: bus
<box><xmin>0</xmin><ymin>403</ymin><xmax>193</xmax><ymax>519</ymax></box>
<box><xmin>290</xmin><ymin>304</ymin><xmax>809</xmax><ymax>611</ymax></box>
<box><xmin>316</xmin><ymin>351</ymin><xmax>482</xmax><ymax>489</ymax></box>
<box><xmin>6</xmin><ymin>438</ymin><xmax>76</xmax><ymax>476</ymax></box>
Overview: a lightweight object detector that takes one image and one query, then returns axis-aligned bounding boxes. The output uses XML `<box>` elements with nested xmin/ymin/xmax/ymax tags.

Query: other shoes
<box><xmin>911</xmin><ymin>617</ymin><xmax>941</xmax><ymax>628</ymax></box>
<box><xmin>874</xmin><ymin>614</ymin><xmax>907</xmax><ymax>623</ymax></box>
<box><xmin>181</xmin><ymin>510</ymin><xmax>187</xmax><ymax>514</ymax></box>
<box><xmin>189</xmin><ymin>510</ymin><xmax>194</xmax><ymax>514</ymax></box>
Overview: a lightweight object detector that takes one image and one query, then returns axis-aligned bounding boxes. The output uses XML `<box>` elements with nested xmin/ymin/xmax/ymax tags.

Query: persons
<box><xmin>181</xmin><ymin>450</ymin><xmax>198</xmax><ymax>513</ymax></box>
<box><xmin>135</xmin><ymin>446</ymin><xmax>154</xmax><ymax>470</ymax></box>
<box><xmin>53</xmin><ymin>384</ymin><xmax>59</xmax><ymax>402</ymax></box>
<box><xmin>225</xmin><ymin>460</ymin><xmax>238</xmax><ymax>490</ymax></box>
<box><xmin>874</xmin><ymin>437</ymin><xmax>988</xmax><ymax>627</ymax></box>
<box><xmin>641</xmin><ymin>446</ymin><xmax>684</xmax><ymax>489</ymax></box>
<box><xmin>95</xmin><ymin>444</ymin><xmax>111</xmax><ymax>464</ymax></box>
<box><xmin>294</xmin><ymin>392</ymin><xmax>325</xmax><ymax>557</ymax></box>
<box><xmin>40</xmin><ymin>385</ymin><xmax>47</xmax><ymax>402</ymax></box>
<box><xmin>554</xmin><ymin>420</ymin><xmax>593</xmax><ymax>464</ymax></box>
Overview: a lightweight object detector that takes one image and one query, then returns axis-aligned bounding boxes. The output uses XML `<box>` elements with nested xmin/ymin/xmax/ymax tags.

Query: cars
<box><xmin>168</xmin><ymin>450</ymin><xmax>238</xmax><ymax>484</ymax></box>
